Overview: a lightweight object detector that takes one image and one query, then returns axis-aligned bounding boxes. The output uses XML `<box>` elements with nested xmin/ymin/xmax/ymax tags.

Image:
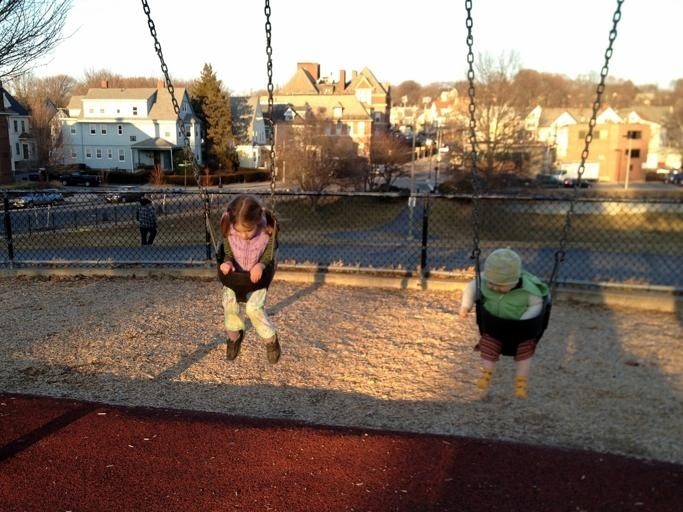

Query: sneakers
<box><xmin>515</xmin><ymin>376</ymin><xmax>529</xmax><ymax>399</ymax></box>
<box><xmin>476</xmin><ymin>368</ymin><xmax>493</xmax><ymax>389</ymax></box>
<box><xmin>227</xmin><ymin>330</ymin><xmax>243</xmax><ymax>360</ymax></box>
<box><xmin>265</xmin><ymin>334</ymin><xmax>280</xmax><ymax>364</ymax></box>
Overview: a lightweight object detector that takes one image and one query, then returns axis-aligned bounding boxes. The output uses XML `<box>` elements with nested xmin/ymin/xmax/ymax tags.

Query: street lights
<box><xmin>405</xmin><ymin>121</ymin><xmax>416</xmax><ymax>193</ymax></box>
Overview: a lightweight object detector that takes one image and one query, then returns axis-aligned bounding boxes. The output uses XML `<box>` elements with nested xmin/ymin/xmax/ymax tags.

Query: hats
<box><xmin>480</xmin><ymin>246</ymin><xmax>522</xmax><ymax>285</ymax></box>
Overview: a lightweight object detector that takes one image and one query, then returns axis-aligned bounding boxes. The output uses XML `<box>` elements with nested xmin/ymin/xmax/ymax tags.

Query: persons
<box><xmin>458</xmin><ymin>247</ymin><xmax>549</xmax><ymax>398</ymax></box>
<box><xmin>217</xmin><ymin>194</ymin><xmax>286</xmax><ymax>365</ymax></box>
<box><xmin>136</xmin><ymin>197</ymin><xmax>157</xmax><ymax>244</ymax></box>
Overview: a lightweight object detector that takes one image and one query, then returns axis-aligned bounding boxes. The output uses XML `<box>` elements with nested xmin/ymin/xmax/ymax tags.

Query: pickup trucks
<box><xmin>58</xmin><ymin>170</ymin><xmax>104</xmax><ymax>187</ymax></box>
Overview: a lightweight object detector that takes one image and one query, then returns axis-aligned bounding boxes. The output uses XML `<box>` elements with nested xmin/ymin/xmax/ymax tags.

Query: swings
<box><xmin>465</xmin><ymin>0</ymin><xmax>626</xmax><ymax>357</ymax></box>
<box><xmin>141</xmin><ymin>0</ymin><xmax>277</xmax><ymax>303</ymax></box>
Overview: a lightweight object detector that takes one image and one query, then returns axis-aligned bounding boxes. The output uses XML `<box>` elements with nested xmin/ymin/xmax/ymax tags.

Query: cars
<box><xmin>641</xmin><ymin>164</ymin><xmax>683</xmax><ymax>187</ymax></box>
<box><xmin>7</xmin><ymin>186</ymin><xmax>63</xmax><ymax>209</ymax></box>
<box><xmin>390</xmin><ymin>126</ymin><xmax>437</xmax><ymax>147</ymax></box>
<box><xmin>104</xmin><ymin>185</ymin><xmax>144</xmax><ymax>203</ymax></box>
<box><xmin>365</xmin><ymin>172</ymin><xmax>599</xmax><ymax>200</ymax></box>
